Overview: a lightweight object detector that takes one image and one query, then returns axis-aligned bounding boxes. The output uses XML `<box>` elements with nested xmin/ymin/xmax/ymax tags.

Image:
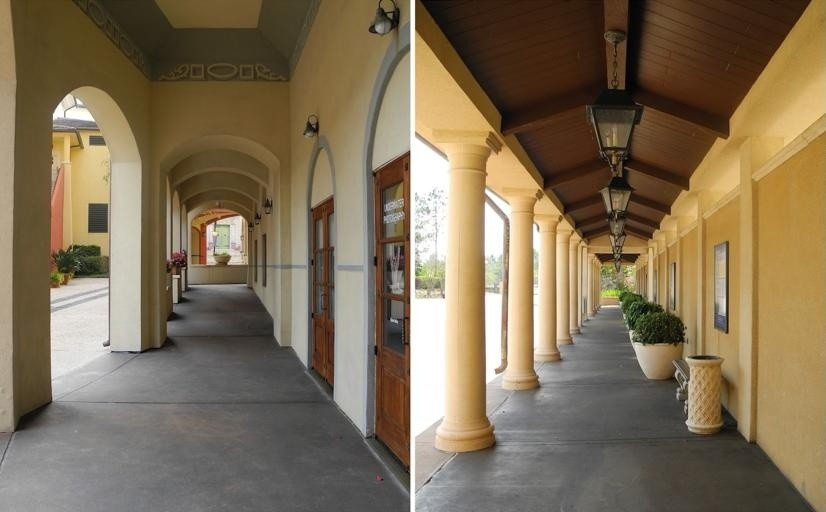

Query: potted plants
<box><xmin>619</xmin><ymin>291</ymin><xmax>687</xmax><ymax>379</ymax></box>
<box><xmin>50</xmin><ymin>272</ymin><xmax>62</xmax><ymax>287</ymax></box>
<box><xmin>51</xmin><ymin>249</ymin><xmax>77</xmax><ymax>285</ymax></box>
<box><xmin>213</xmin><ymin>253</ymin><xmax>231</xmax><ymax>263</ymax></box>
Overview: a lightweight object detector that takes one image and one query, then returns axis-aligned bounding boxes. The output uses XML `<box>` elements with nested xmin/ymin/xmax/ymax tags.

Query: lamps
<box><xmin>302</xmin><ymin>114</ymin><xmax>319</xmax><ymax>137</ymax></box>
<box><xmin>586</xmin><ymin>30</ymin><xmax>643</xmax><ymax>272</ymax></box>
<box><xmin>369</xmin><ymin>0</ymin><xmax>400</xmax><ymax>35</ymax></box>
<box><xmin>248</xmin><ymin>198</ymin><xmax>272</xmax><ymax>231</ymax></box>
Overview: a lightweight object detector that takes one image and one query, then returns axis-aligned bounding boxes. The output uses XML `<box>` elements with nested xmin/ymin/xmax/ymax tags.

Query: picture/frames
<box><xmin>713</xmin><ymin>241</ymin><xmax>729</xmax><ymax>334</ymax></box>
<box><xmin>644</xmin><ymin>263</ymin><xmax>676</xmax><ymax>311</ymax></box>
